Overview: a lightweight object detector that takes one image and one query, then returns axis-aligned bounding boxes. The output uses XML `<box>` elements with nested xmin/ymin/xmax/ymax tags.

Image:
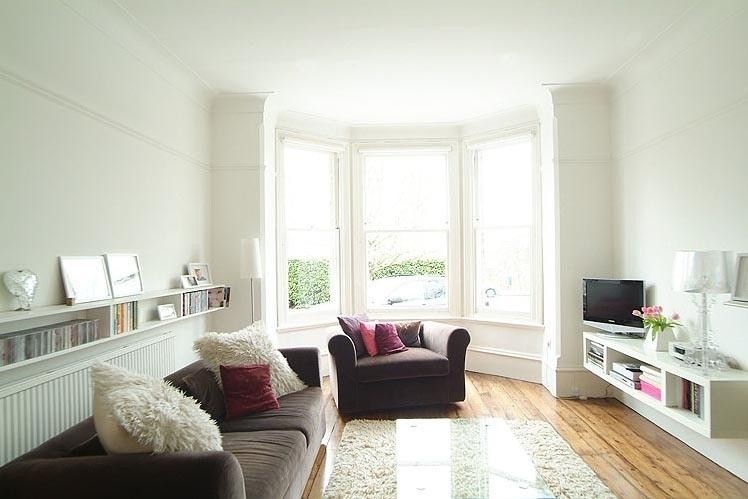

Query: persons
<box><xmin>194</xmin><ymin>269</ymin><xmax>207</xmax><ymax>281</ymax></box>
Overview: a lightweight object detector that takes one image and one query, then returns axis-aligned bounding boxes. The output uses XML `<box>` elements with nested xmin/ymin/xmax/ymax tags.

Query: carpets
<box><xmin>321</xmin><ymin>415</ymin><xmax>620</xmax><ymax>498</ymax></box>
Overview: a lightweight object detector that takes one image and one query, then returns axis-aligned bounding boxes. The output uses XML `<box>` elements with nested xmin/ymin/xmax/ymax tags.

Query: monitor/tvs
<box><xmin>583</xmin><ymin>278</ymin><xmax>647</xmax><ymax>340</ymax></box>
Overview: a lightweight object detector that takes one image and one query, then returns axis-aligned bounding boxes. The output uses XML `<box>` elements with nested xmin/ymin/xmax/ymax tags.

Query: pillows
<box><xmin>334</xmin><ymin>312</ymin><xmax>422</xmax><ymax>357</ymax></box>
<box><xmin>218</xmin><ymin>362</ymin><xmax>281</xmax><ymax>422</ymax></box>
<box><xmin>190</xmin><ymin>317</ymin><xmax>311</xmax><ymax>399</ymax></box>
<box><xmin>80</xmin><ymin>352</ymin><xmax>227</xmax><ymax>455</ymax></box>
<box><xmin>179</xmin><ymin>364</ymin><xmax>227</xmax><ymax>425</ymax></box>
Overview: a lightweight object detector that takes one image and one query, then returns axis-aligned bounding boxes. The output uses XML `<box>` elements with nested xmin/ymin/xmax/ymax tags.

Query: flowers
<box><xmin>625</xmin><ymin>304</ymin><xmax>684</xmax><ymax>341</ymax></box>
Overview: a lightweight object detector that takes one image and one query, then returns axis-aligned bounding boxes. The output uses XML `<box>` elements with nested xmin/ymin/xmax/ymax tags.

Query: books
<box><xmin>112</xmin><ymin>301</ymin><xmax>139</xmax><ymax>335</ymax></box>
<box><xmin>679</xmin><ymin>377</ymin><xmax>704</xmax><ymax>420</ymax></box>
<box><xmin>586</xmin><ymin>341</ymin><xmax>604</xmax><ymax>369</ymax></box>
<box><xmin>0</xmin><ymin>317</ymin><xmax>101</xmax><ymax>367</ymax></box>
<box><xmin>183</xmin><ymin>287</ymin><xmax>226</xmax><ymax>317</ymax></box>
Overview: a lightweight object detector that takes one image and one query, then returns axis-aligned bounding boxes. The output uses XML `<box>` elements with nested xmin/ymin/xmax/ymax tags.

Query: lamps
<box><xmin>238</xmin><ymin>235</ymin><xmax>264</xmax><ymax>326</ymax></box>
<box><xmin>684</xmin><ymin>250</ymin><xmax>733</xmax><ymax>377</ymax></box>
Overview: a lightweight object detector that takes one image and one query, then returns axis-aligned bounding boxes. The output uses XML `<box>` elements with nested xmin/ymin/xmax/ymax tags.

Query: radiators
<box><xmin>0</xmin><ymin>329</ymin><xmax>176</xmax><ymax>467</ymax></box>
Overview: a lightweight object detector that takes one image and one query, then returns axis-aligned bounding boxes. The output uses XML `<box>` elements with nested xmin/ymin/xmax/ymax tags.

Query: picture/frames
<box><xmin>54</xmin><ymin>249</ymin><xmax>212</xmax><ymax>305</ymax></box>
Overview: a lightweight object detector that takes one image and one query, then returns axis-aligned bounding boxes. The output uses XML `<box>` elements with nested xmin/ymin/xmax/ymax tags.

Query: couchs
<box><xmin>0</xmin><ymin>347</ymin><xmax>327</xmax><ymax>499</ymax></box>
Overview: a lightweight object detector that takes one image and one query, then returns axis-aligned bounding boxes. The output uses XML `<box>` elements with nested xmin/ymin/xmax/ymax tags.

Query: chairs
<box><xmin>327</xmin><ymin>320</ymin><xmax>472</xmax><ymax>416</ymax></box>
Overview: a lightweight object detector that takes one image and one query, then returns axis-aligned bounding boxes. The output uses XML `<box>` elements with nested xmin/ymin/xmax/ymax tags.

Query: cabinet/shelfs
<box><xmin>0</xmin><ymin>283</ymin><xmax>226</xmax><ymax>374</ymax></box>
<box><xmin>581</xmin><ymin>332</ymin><xmax>747</xmax><ymax>440</ymax></box>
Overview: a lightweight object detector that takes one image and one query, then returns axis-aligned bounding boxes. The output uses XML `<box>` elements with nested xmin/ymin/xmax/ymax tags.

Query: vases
<box><xmin>656</xmin><ymin>327</ymin><xmax>679</xmax><ymax>352</ymax></box>
